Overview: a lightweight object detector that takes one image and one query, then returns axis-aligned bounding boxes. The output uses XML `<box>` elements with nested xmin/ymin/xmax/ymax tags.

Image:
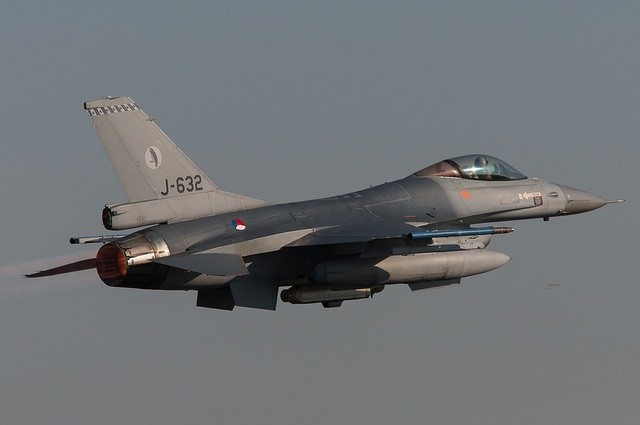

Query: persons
<box><xmin>472</xmin><ymin>156</ymin><xmax>493</xmax><ymax>181</ymax></box>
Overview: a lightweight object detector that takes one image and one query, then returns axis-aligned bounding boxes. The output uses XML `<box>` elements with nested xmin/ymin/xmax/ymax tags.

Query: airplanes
<box><xmin>23</xmin><ymin>95</ymin><xmax>628</xmax><ymax>311</ymax></box>
